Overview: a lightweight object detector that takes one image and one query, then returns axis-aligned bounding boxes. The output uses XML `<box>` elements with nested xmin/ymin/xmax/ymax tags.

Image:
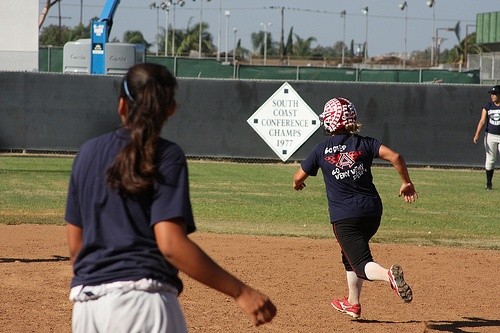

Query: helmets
<box><xmin>321</xmin><ymin>96</ymin><xmax>359</xmax><ymax>133</ymax></box>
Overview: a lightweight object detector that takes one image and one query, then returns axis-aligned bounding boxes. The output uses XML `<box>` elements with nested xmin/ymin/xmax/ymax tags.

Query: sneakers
<box><xmin>331</xmin><ymin>295</ymin><xmax>362</xmax><ymax>318</ymax></box>
<box><xmin>388</xmin><ymin>264</ymin><xmax>413</xmax><ymax>303</ymax></box>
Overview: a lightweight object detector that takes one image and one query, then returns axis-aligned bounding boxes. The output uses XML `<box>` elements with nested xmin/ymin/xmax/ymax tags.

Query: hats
<box><xmin>486</xmin><ymin>84</ymin><xmax>500</xmax><ymax>94</ymax></box>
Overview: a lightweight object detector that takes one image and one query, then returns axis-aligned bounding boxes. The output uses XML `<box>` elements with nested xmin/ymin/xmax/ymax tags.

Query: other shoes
<box><xmin>484</xmin><ymin>185</ymin><xmax>492</xmax><ymax>190</ymax></box>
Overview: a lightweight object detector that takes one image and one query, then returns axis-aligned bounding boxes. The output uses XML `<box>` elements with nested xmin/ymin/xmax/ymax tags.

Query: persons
<box><xmin>472</xmin><ymin>85</ymin><xmax>500</xmax><ymax>189</ymax></box>
<box><xmin>293</xmin><ymin>97</ymin><xmax>418</xmax><ymax>317</ymax></box>
<box><xmin>63</xmin><ymin>63</ymin><xmax>278</xmax><ymax>332</ymax></box>
